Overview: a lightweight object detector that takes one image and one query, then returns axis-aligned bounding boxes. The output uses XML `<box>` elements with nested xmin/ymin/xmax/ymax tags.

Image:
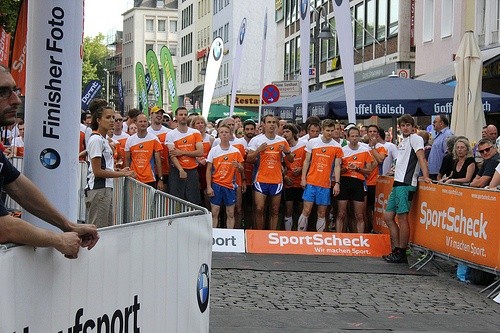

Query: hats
<box><xmin>344</xmin><ymin>124</ymin><xmax>356</xmax><ymax>131</ymax></box>
<box><xmin>150</xmin><ymin>106</ymin><xmax>165</xmax><ymax>113</ymax></box>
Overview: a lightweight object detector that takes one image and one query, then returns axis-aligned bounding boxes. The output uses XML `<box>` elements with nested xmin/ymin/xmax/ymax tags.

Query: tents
<box><xmin>185</xmin><ymin>99</ymin><xmax>258</xmax><ymax>123</ymax></box>
<box><xmin>260</xmin><ymin>68</ymin><xmax>499</xmax><ymax>125</ymax></box>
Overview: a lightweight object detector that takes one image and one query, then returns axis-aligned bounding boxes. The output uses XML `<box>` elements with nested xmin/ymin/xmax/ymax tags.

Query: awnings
<box><xmin>413</xmin><ymin>47</ymin><xmax>500</xmax><ymax>84</ymax></box>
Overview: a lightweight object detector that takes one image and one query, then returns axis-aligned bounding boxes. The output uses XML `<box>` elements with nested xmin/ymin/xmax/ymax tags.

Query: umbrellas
<box><xmin>450</xmin><ymin>30</ymin><xmax>487</xmax><ymax>149</ymax></box>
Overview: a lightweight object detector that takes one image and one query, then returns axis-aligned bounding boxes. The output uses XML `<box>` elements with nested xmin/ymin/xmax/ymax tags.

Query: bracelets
<box><xmin>495</xmin><ymin>185</ymin><xmax>500</xmax><ymax>190</ymax></box>
<box><xmin>356</xmin><ymin>167</ymin><xmax>360</xmax><ymax>172</ymax></box>
<box><xmin>451</xmin><ymin>178</ymin><xmax>454</xmax><ymax>183</ymax></box>
<box><xmin>335</xmin><ymin>181</ymin><xmax>341</xmax><ymax>184</ymax></box>
<box><xmin>238</xmin><ymin>165</ymin><xmax>240</xmax><ymax>170</ymax></box>
<box><xmin>116</xmin><ymin>168</ymin><xmax>119</xmax><ymax>171</ymax></box>
<box><xmin>158</xmin><ymin>176</ymin><xmax>165</xmax><ymax>181</ymax></box>
<box><xmin>284</xmin><ymin>151</ymin><xmax>292</xmax><ymax>155</ymax></box>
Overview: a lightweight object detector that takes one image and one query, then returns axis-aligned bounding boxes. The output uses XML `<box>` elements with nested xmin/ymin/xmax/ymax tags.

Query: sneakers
<box><xmin>382</xmin><ymin>251</ymin><xmax>407</xmax><ymax>263</ymax></box>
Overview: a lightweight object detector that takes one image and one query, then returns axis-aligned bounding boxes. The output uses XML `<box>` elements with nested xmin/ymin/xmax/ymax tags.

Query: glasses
<box><xmin>479</xmin><ymin>145</ymin><xmax>493</xmax><ymax>154</ymax></box>
<box><xmin>0</xmin><ymin>88</ymin><xmax>22</xmax><ymax>98</ymax></box>
<box><xmin>115</xmin><ymin>118</ymin><xmax>123</xmax><ymax>122</ymax></box>
<box><xmin>433</xmin><ymin>120</ymin><xmax>437</xmax><ymax>123</ymax></box>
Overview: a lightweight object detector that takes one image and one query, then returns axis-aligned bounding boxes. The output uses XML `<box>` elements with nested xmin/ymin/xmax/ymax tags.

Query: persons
<box><xmin>417</xmin><ymin>130</ymin><xmax>432</xmax><ymax>177</ymax></box>
<box><xmin>422</xmin><ymin>113</ymin><xmax>454</xmax><ymax>180</ymax></box>
<box><xmin>0</xmin><ymin>97</ymin><xmax>424</xmax><ymax>233</ymax></box>
<box><xmin>432</xmin><ymin>123</ymin><xmax>500</xmax><ymax>190</ymax></box>
<box><xmin>381</xmin><ymin>112</ymin><xmax>433</xmax><ymax>264</ymax></box>
<box><xmin>0</xmin><ymin>62</ymin><xmax>101</xmax><ymax>260</ymax></box>
